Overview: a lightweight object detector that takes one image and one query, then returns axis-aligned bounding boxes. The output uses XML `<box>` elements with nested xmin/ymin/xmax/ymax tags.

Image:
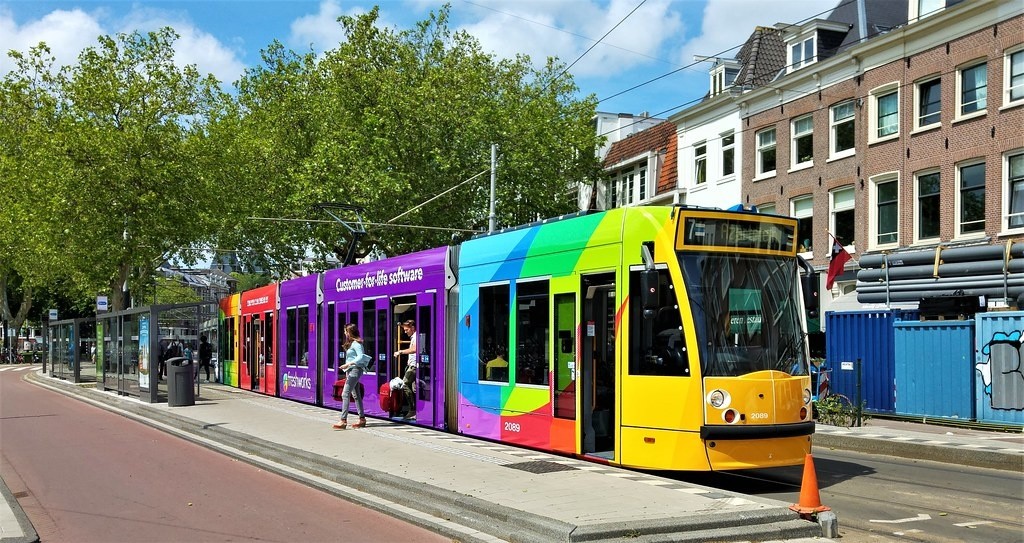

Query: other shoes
<box><xmin>332</xmin><ymin>420</ymin><xmax>347</xmax><ymax>431</ymax></box>
<box><xmin>204</xmin><ymin>380</ymin><xmax>210</xmax><ymax>383</ymax></box>
<box><xmin>353</xmin><ymin>419</ymin><xmax>367</xmax><ymax>428</ymax></box>
<box><xmin>193</xmin><ymin>378</ymin><xmax>198</xmax><ymax>382</ymax></box>
<box><xmin>406</xmin><ymin>411</ymin><xmax>417</xmax><ymax>419</ymax></box>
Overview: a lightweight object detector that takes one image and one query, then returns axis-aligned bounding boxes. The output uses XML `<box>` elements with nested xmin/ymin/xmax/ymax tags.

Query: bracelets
<box><xmin>399</xmin><ymin>351</ymin><xmax>402</xmax><ymax>355</ymax></box>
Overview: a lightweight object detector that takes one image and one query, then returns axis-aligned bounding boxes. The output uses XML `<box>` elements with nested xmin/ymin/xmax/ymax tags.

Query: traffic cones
<box><xmin>788</xmin><ymin>452</ymin><xmax>830</xmax><ymax>514</ymax></box>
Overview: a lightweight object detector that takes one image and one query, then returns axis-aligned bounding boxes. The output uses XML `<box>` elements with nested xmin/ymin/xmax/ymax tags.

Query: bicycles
<box><xmin>809</xmin><ymin>367</ymin><xmax>855</xmax><ymax>429</ymax></box>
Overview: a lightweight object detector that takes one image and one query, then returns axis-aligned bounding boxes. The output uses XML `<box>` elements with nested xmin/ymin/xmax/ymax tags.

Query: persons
<box><xmin>267</xmin><ymin>345</ymin><xmax>271</xmax><ymax>359</ymax></box>
<box><xmin>483</xmin><ymin>335</ymin><xmax>545</xmax><ymax>384</ymax></box>
<box><xmin>332</xmin><ymin>323</ymin><xmax>372</xmax><ymax>430</ymax></box>
<box><xmin>394</xmin><ymin>320</ymin><xmax>422</xmax><ymax>418</ymax></box>
<box><xmin>1</xmin><ymin>339</ymin><xmax>39</xmax><ymax>364</ymax></box>
<box><xmin>300</xmin><ymin>352</ymin><xmax>308</xmax><ymax>365</ymax></box>
<box><xmin>106</xmin><ymin>348</ymin><xmax>111</xmax><ymax>372</ymax></box>
<box><xmin>194</xmin><ymin>335</ymin><xmax>213</xmax><ymax>383</ymax></box>
<box><xmin>158</xmin><ymin>335</ymin><xmax>194</xmax><ymax>380</ymax></box>
<box><xmin>90</xmin><ymin>343</ymin><xmax>96</xmax><ymax>364</ymax></box>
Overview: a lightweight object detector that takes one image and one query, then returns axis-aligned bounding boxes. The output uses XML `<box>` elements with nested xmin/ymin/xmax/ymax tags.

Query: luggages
<box><xmin>380</xmin><ymin>352</ymin><xmax>404</xmax><ymax>412</ymax></box>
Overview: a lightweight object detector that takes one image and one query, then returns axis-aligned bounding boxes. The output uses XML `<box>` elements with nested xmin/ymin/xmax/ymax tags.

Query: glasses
<box><xmin>345</xmin><ymin>323</ymin><xmax>353</xmax><ymax>332</ymax></box>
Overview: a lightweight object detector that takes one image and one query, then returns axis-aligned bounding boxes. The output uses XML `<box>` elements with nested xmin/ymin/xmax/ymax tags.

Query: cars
<box><xmin>210</xmin><ymin>352</ymin><xmax>217</xmax><ymax>366</ymax></box>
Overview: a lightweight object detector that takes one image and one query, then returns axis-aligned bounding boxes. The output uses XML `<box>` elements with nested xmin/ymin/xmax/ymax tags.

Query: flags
<box><xmin>827</xmin><ymin>239</ymin><xmax>852</xmax><ymax>290</ymax></box>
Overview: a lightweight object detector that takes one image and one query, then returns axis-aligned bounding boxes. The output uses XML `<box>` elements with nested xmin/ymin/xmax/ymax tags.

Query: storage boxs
<box><xmin>891</xmin><ymin>317</ymin><xmax>978</xmax><ymax>423</ymax></box>
<box><xmin>974</xmin><ymin>310</ymin><xmax>1024</xmax><ymax>426</ymax></box>
<box><xmin>824</xmin><ymin>308</ymin><xmax>920</xmax><ymax>415</ymax></box>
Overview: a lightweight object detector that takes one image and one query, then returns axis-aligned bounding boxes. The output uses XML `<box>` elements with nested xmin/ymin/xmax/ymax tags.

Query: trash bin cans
<box><xmin>167</xmin><ymin>357</ymin><xmax>196</xmax><ymax>406</ymax></box>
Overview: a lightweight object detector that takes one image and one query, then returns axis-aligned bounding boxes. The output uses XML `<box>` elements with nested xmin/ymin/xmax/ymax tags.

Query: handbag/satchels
<box><xmin>331</xmin><ymin>377</ymin><xmax>365</xmax><ymax>402</ymax></box>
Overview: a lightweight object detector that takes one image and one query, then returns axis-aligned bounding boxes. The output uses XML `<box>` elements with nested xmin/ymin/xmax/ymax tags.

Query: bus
<box><xmin>217</xmin><ymin>202</ymin><xmax>819</xmax><ymax>473</ymax></box>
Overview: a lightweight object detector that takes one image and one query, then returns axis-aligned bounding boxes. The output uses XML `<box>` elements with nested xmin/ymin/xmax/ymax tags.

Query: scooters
<box><xmin>0</xmin><ymin>347</ymin><xmax>26</xmax><ymax>365</ymax></box>
<box><xmin>32</xmin><ymin>350</ymin><xmax>40</xmax><ymax>363</ymax></box>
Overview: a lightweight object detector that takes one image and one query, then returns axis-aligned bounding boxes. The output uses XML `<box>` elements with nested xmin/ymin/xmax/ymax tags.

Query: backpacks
<box><xmin>164</xmin><ymin>344</ymin><xmax>182</xmax><ymax>365</ymax></box>
